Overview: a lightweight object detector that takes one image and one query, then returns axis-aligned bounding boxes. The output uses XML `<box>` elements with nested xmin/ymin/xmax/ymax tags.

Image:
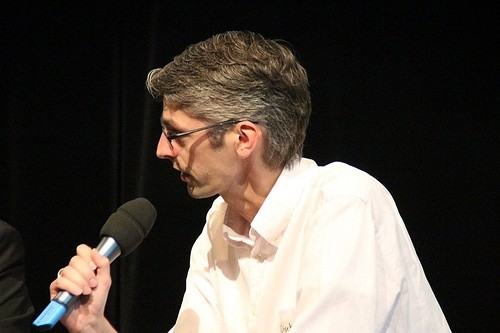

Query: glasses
<box><xmin>152</xmin><ymin>115</ymin><xmax>258</xmax><ymax>147</ymax></box>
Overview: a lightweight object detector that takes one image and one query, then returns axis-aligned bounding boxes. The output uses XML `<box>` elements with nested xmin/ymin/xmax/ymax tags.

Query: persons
<box><xmin>33</xmin><ymin>30</ymin><xmax>454</xmax><ymax>333</ymax></box>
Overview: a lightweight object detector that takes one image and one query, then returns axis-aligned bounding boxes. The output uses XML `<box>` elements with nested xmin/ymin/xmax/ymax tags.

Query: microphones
<box><xmin>32</xmin><ymin>198</ymin><xmax>157</xmax><ymax>330</ymax></box>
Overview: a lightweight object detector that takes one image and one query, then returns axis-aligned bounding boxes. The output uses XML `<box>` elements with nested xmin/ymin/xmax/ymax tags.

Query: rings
<box><xmin>57</xmin><ymin>268</ymin><xmax>64</xmax><ymax>278</ymax></box>
